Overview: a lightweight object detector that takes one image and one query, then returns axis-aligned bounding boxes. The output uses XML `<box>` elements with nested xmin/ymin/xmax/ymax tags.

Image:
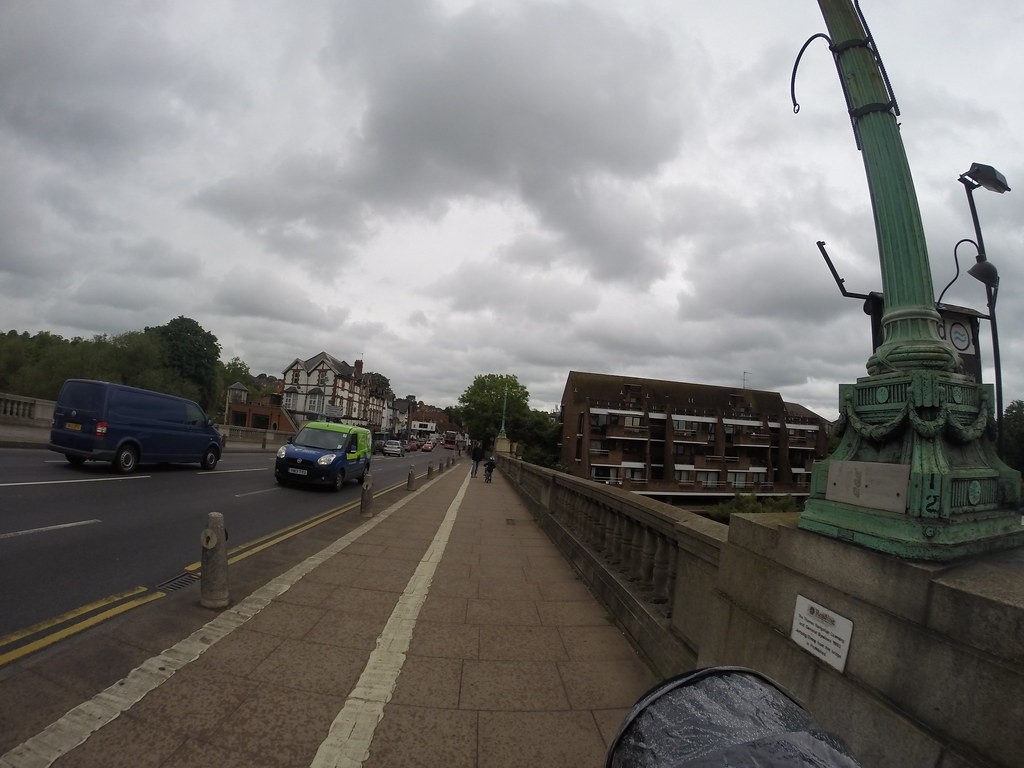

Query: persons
<box><xmin>351</xmin><ymin>438</ymin><xmax>356</xmax><ymax>450</ymax></box>
<box><xmin>471</xmin><ymin>441</ymin><xmax>482</xmax><ymax>478</ymax></box>
<box><xmin>483</xmin><ymin>456</ymin><xmax>496</xmax><ymax>483</ymax></box>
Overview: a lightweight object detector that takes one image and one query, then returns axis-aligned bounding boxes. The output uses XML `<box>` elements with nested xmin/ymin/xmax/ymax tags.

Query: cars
<box><xmin>371</xmin><ymin>442</ymin><xmax>377</xmax><ymax>455</ymax></box>
<box><xmin>400</xmin><ymin>437</ymin><xmax>446</xmax><ymax>453</ymax></box>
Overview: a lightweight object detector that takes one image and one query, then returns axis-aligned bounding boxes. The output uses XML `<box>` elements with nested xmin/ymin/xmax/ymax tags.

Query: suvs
<box><xmin>383</xmin><ymin>439</ymin><xmax>406</xmax><ymax>457</ymax></box>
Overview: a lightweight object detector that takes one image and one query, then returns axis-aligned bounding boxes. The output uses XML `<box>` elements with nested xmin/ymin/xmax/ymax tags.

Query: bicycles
<box><xmin>484</xmin><ymin>466</ymin><xmax>491</xmax><ymax>484</ymax></box>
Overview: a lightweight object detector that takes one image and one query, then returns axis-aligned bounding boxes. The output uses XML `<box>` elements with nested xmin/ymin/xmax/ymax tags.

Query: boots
<box><xmin>471</xmin><ymin>472</ymin><xmax>474</xmax><ymax>478</ymax></box>
<box><xmin>474</xmin><ymin>472</ymin><xmax>477</xmax><ymax>478</ymax></box>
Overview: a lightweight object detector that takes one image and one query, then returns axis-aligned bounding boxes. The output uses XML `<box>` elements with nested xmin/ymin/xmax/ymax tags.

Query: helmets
<box><xmin>489</xmin><ymin>456</ymin><xmax>495</xmax><ymax>461</ymax></box>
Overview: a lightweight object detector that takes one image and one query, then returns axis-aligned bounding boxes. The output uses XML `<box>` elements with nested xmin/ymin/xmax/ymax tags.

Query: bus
<box><xmin>373</xmin><ymin>431</ymin><xmax>391</xmax><ymax>453</ymax></box>
<box><xmin>443</xmin><ymin>430</ymin><xmax>458</xmax><ymax>450</ymax></box>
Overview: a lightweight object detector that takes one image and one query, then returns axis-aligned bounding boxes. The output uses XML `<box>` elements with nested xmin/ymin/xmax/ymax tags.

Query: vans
<box><xmin>274</xmin><ymin>421</ymin><xmax>373</xmax><ymax>493</ymax></box>
<box><xmin>47</xmin><ymin>379</ymin><xmax>225</xmax><ymax>475</ymax></box>
